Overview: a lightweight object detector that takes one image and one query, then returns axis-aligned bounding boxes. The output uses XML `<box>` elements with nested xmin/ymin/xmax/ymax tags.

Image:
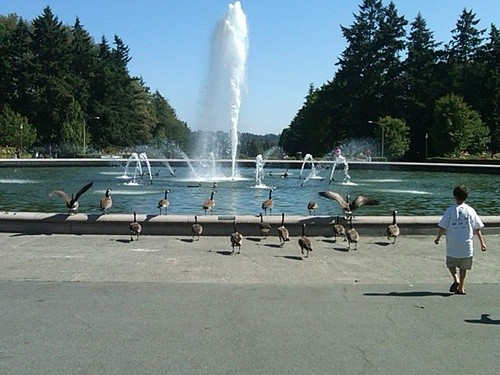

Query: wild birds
<box><xmin>201</xmin><ymin>189</ymin><xmax>217</xmax><ymax>212</ymax></box>
<box><xmin>192</xmin><ymin>214</ymin><xmax>203</xmax><ymax>241</ymax></box>
<box><xmin>100</xmin><ymin>188</ymin><xmax>113</xmax><ymax>214</ymax></box>
<box><xmin>387</xmin><ymin>210</ymin><xmax>400</xmax><ymax>244</ymax></box>
<box><xmin>333</xmin><ymin>214</ymin><xmax>360</xmax><ymax>249</ymax></box>
<box><xmin>129</xmin><ymin>210</ymin><xmax>141</xmax><ymax>240</ymax></box>
<box><xmin>261</xmin><ymin>188</ymin><xmax>273</xmax><ymax>212</ymax></box>
<box><xmin>319</xmin><ymin>189</ymin><xmax>381</xmax><ymax>214</ymax></box>
<box><xmin>48</xmin><ymin>182</ymin><xmax>97</xmax><ymax>214</ymax></box>
<box><xmin>230</xmin><ymin>220</ymin><xmax>243</xmax><ymax>253</ymax></box>
<box><xmin>157</xmin><ymin>190</ymin><xmax>168</xmax><ymax>214</ymax></box>
<box><xmin>259</xmin><ymin>202</ymin><xmax>319</xmax><ymax>258</ymax></box>
<box><xmin>136</xmin><ymin>169</ymin><xmax>290</xmax><ymax>189</ymax></box>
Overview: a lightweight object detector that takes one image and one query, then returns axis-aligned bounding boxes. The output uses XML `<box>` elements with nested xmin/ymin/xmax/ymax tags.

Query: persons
<box><xmin>434</xmin><ymin>185</ymin><xmax>488</xmax><ymax>294</ymax></box>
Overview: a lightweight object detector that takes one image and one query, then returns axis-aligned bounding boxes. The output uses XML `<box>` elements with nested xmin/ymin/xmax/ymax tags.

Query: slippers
<box><xmin>449</xmin><ymin>282</ymin><xmax>460</xmax><ymax>292</ymax></box>
<box><xmin>455</xmin><ymin>289</ymin><xmax>466</xmax><ymax>294</ymax></box>
